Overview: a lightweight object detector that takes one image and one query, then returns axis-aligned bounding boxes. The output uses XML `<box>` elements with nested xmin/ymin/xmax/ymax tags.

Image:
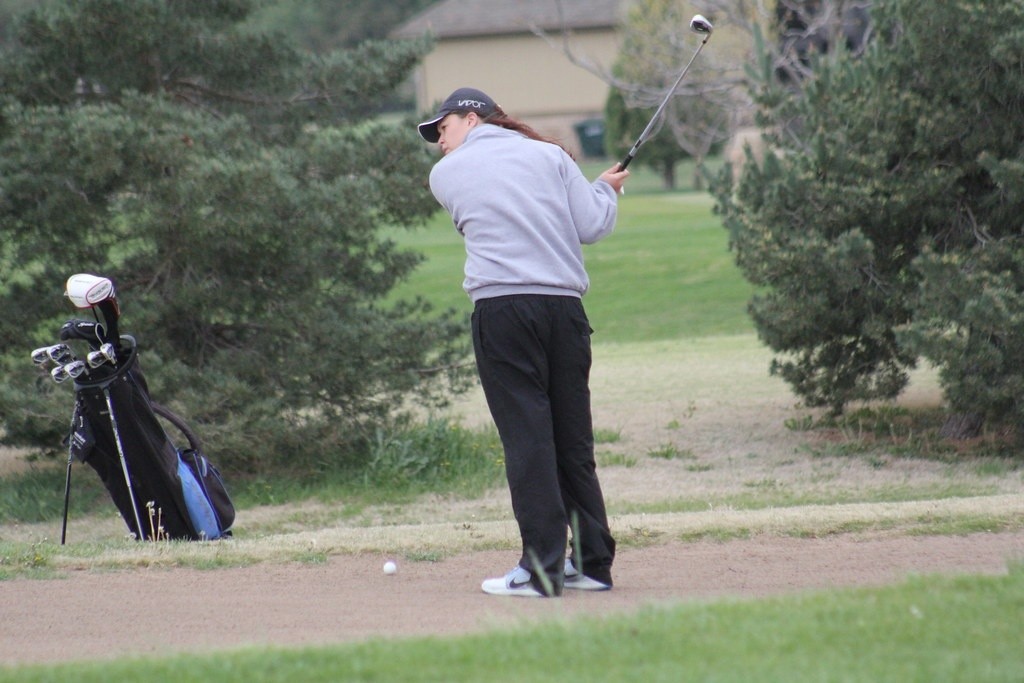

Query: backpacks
<box><xmin>175</xmin><ymin>444</ymin><xmax>238</xmax><ymax>541</ymax></box>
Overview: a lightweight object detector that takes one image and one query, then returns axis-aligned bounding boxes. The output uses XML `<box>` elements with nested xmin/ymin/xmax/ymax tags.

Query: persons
<box><xmin>417</xmin><ymin>87</ymin><xmax>630</xmax><ymax>596</ymax></box>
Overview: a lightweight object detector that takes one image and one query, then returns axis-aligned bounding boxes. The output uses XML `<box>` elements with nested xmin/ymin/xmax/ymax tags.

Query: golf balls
<box><xmin>382</xmin><ymin>560</ymin><xmax>397</xmax><ymax>575</ymax></box>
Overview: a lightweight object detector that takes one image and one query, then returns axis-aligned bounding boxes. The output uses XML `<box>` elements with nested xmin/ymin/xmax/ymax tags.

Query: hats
<box><xmin>418</xmin><ymin>87</ymin><xmax>504</xmax><ymax>143</ymax></box>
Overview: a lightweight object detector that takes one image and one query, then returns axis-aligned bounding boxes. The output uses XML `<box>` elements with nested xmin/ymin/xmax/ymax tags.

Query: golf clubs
<box><xmin>30</xmin><ymin>342</ymin><xmax>120</xmax><ymax>384</ymax></box>
<box><xmin>617</xmin><ymin>13</ymin><xmax>715</xmax><ymax>172</ymax></box>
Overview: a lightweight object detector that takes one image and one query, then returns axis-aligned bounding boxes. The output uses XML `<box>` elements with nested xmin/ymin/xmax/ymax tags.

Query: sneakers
<box><xmin>481</xmin><ymin>566</ymin><xmax>542</xmax><ymax>595</ymax></box>
<box><xmin>564</xmin><ymin>556</ymin><xmax>608</xmax><ymax>589</ymax></box>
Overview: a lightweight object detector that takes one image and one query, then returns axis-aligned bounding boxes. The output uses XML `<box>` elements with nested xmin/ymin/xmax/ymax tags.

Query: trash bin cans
<box><xmin>570</xmin><ymin>118</ymin><xmax>605</xmax><ymax>160</ymax></box>
